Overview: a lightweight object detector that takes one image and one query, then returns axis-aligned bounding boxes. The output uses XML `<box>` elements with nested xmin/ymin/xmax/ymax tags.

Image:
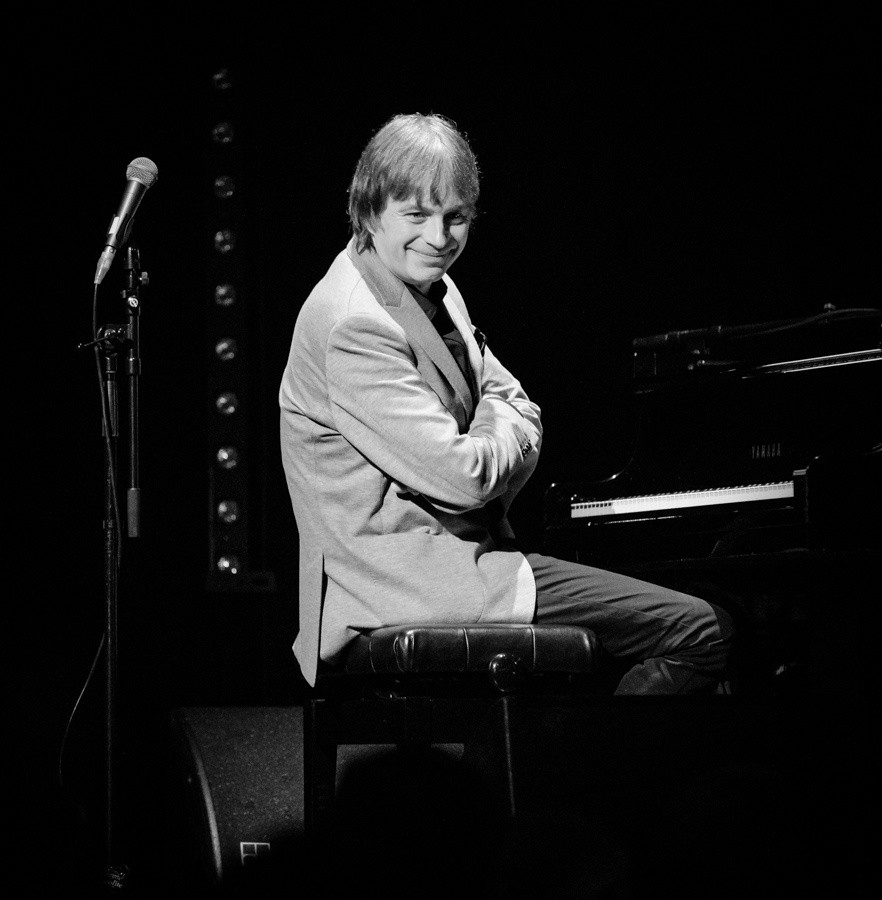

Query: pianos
<box><xmin>512</xmin><ymin>300</ymin><xmax>882</xmax><ymax>696</ymax></box>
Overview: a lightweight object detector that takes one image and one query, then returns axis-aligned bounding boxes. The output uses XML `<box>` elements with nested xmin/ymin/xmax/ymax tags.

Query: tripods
<box><xmin>80</xmin><ymin>246</ymin><xmax>144</xmax><ymax>900</ymax></box>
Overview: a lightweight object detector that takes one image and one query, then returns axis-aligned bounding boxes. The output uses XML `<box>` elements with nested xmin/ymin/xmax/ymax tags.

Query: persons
<box><xmin>278</xmin><ymin>113</ymin><xmax>741</xmax><ymax>697</ymax></box>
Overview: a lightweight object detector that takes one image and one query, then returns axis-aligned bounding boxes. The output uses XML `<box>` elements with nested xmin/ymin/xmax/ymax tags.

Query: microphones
<box><xmin>92</xmin><ymin>156</ymin><xmax>160</xmax><ymax>286</ymax></box>
<box><xmin>632</xmin><ymin>327</ymin><xmax>719</xmax><ymax>352</ymax></box>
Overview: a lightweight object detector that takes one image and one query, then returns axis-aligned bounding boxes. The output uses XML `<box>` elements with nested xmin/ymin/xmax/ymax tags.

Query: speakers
<box><xmin>162</xmin><ymin>704</ymin><xmax>480</xmax><ymax>893</ymax></box>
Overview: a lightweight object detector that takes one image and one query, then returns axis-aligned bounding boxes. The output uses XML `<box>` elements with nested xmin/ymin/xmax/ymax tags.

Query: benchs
<box><xmin>299</xmin><ymin>623</ymin><xmax>627</xmax><ymax>900</ymax></box>
